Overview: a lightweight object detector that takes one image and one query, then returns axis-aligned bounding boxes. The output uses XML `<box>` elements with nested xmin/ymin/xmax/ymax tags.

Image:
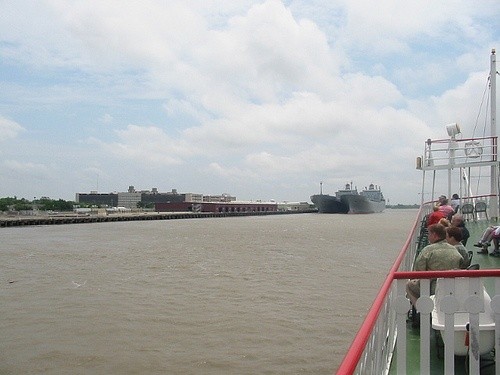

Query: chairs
<box><xmin>460</xmin><ymin>204</ymin><xmax>474</xmax><ymax>223</ymax></box>
<box><xmin>474</xmin><ymin>202</ymin><xmax>489</xmax><ymax>221</ymax></box>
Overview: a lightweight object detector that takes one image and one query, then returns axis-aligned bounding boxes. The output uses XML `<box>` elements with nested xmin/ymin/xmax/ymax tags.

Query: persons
<box><xmin>429</xmin><ymin>194</ymin><xmax>500</xmax><ymax>264</ymax></box>
<box><xmin>406</xmin><ymin>223</ymin><xmax>464</xmax><ymax>328</ymax></box>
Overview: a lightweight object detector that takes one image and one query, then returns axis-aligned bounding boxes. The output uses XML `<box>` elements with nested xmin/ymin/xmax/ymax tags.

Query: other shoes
<box><xmin>474</xmin><ymin>243</ymin><xmax>482</xmax><ymax>247</ymax></box>
<box><xmin>489</xmin><ymin>248</ymin><xmax>500</xmax><ymax>256</ymax></box>
<box><xmin>412</xmin><ymin>312</ymin><xmax>420</xmax><ymax>328</ymax></box>
<box><xmin>476</xmin><ymin>248</ymin><xmax>488</xmax><ymax>254</ymax></box>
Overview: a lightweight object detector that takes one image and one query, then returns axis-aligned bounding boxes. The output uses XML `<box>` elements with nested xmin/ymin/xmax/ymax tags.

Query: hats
<box><xmin>439</xmin><ymin>194</ymin><xmax>446</xmax><ymax>198</ymax></box>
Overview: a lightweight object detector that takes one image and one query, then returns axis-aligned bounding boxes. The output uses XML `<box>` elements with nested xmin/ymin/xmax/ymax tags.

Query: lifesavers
<box><xmin>465</xmin><ymin>141</ymin><xmax>483</xmax><ymax>158</ymax></box>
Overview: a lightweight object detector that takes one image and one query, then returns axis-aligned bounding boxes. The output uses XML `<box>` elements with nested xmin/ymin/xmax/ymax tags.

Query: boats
<box><xmin>345</xmin><ymin>184</ymin><xmax>386</xmax><ymax>214</ymax></box>
<box><xmin>309</xmin><ymin>181</ymin><xmax>358</xmax><ymax>214</ymax></box>
